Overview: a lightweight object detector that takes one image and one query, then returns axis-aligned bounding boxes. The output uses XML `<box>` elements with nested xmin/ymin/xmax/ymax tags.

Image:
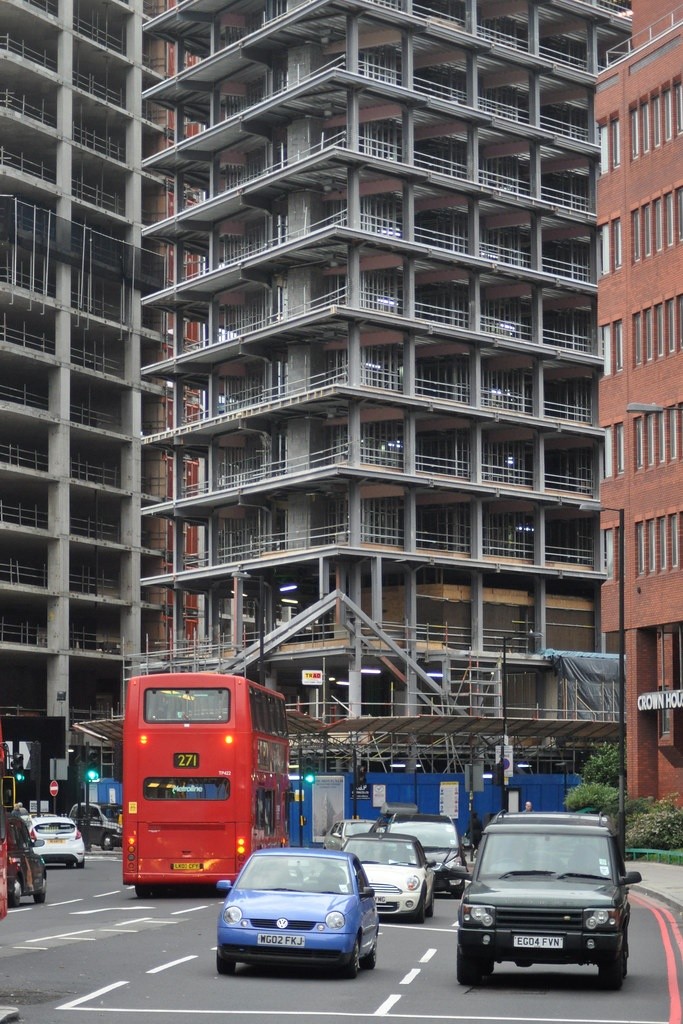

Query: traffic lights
<box><xmin>13</xmin><ymin>753</ymin><xmax>24</xmax><ymax>782</ymax></box>
<box><xmin>358</xmin><ymin>765</ymin><xmax>367</xmax><ymax>786</ymax></box>
<box><xmin>87</xmin><ymin>749</ymin><xmax>98</xmax><ymax>781</ymax></box>
<box><xmin>303</xmin><ymin>755</ymin><xmax>314</xmax><ymax>784</ymax></box>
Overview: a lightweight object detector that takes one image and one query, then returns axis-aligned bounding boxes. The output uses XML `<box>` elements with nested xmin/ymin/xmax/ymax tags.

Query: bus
<box><xmin>122</xmin><ymin>675</ymin><xmax>295</xmax><ymax>897</ymax></box>
<box><xmin>0</xmin><ymin>723</ymin><xmax>19</xmax><ymax>920</ymax></box>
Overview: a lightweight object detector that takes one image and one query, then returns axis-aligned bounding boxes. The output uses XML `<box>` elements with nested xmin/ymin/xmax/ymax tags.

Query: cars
<box><xmin>385</xmin><ymin>813</ymin><xmax>465</xmax><ymax>896</ymax></box>
<box><xmin>341</xmin><ymin>833</ymin><xmax>437</xmax><ymax>924</ymax></box>
<box><xmin>216</xmin><ymin>846</ymin><xmax>379</xmax><ymax>978</ymax></box>
<box><xmin>26</xmin><ymin>813</ymin><xmax>57</xmax><ymax>825</ymax></box>
<box><xmin>25</xmin><ymin>817</ymin><xmax>85</xmax><ymax>868</ymax></box>
<box><xmin>370</xmin><ymin>801</ymin><xmax>418</xmax><ymax>836</ymax></box>
<box><xmin>324</xmin><ymin>819</ymin><xmax>376</xmax><ymax>851</ymax></box>
<box><xmin>7</xmin><ymin>816</ymin><xmax>47</xmax><ymax>908</ymax></box>
<box><xmin>68</xmin><ymin>802</ymin><xmax>123</xmax><ymax>851</ymax></box>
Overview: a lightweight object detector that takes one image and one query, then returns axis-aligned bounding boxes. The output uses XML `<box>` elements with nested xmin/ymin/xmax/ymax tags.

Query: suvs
<box><xmin>449</xmin><ymin>810</ymin><xmax>643</xmax><ymax>990</ymax></box>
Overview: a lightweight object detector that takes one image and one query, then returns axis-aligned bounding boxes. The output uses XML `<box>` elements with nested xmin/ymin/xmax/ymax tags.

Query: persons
<box><xmin>521</xmin><ymin>802</ymin><xmax>535</xmax><ymax>812</ymax></box>
<box><xmin>466</xmin><ymin>812</ymin><xmax>484</xmax><ymax>861</ymax></box>
<box><xmin>11</xmin><ymin>802</ymin><xmax>28</xmax><ymax>816</ymax></box>
<box><xmin>392</xmin><ymin>844</ymin><xmax>411</xmax><ymax>864</ymax></box>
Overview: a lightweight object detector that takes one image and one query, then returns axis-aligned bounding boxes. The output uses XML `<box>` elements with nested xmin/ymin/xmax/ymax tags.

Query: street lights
<box><xmin>466</xmin><ymin>763</ymin><xmax>482</xmax><ymax>860</ymax></box>
<box><xmin>580</xmin><ymin>502</ymin><xmax>624</xmax><ymax>859</ymax></box>
<box><xmin>501</xmin><ymin>632</ymin><xmax>544</xmax><ymax>811</ymax></box>
<box><xmin>554</xmin><ymin>762</ymin><xmax>568</xmax><ymax>812</ymax></box>
<box><xmin>231</xmin><ymin>571</ymin><xmax>264</xmax><ymax>685</ymax></box>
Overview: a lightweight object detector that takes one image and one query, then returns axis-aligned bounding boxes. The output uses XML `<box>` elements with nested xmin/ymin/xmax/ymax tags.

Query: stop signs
<box><xmin>49</xmin><ymin>780</ymin><xmax>59</xmax><ymax>797</ymax></box>
